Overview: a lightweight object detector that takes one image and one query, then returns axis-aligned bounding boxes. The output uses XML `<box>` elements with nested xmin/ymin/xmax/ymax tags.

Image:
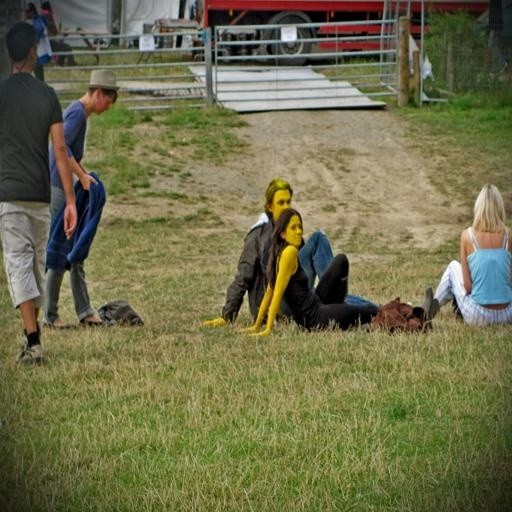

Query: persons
<box><xmin>201</xmin><ymin>177</ymin><xmax>375</xmax><ymax>330</ymax></box>
<box><xmin>42</xmin><ymin>2</ymin><xmax>76</xmax><ymax>68</ymax></box>
<box><xmin>0</xmin><ymin>19</ymin><xmax>81</xmax><ymax>363</ymax></box>
<box><xmin>38</xmin><ymin>67</ymin><xmax>124</xmax><ymax>327</ymax></box>
<box><xmin>237</xmin><ymin>207</ymin><xmax>441</xmax><ymax>340</ymax></box>
<box><xmin>433</xmin><ymin>182</ymin><xmax>511</xmax><ymax>327</ymax></box>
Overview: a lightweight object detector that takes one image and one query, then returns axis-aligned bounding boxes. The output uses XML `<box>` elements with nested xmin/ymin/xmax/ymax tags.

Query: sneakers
<box><xmin>83</xmin><ymin>318</ymin><xmax>113</xmax><ymax>327</ymax></box>
<box><xmin>43</xmin><ymin>319</ymin><xmax>76</xmax><ymax>329</ymax></box>
<box><xmin>15</xmin><ymin>322</ymin><xmax>44</xmax><ymax>362</ymax></box>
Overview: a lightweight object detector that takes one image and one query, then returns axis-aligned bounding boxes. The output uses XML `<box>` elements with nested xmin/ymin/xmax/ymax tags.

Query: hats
<box><xmin>267</xmin><ymin>177</ymin><xmax>291</xmax><ymax>201</ymax></box>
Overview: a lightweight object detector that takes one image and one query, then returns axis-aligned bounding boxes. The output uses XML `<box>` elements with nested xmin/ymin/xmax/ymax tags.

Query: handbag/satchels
<box><xmin>98</xmin><ymin>301</ymin><xmax>144</xmax><ymax>326</ymax></box>
<box><xmin>374</xmin><ymin>297</ymin><xmax>421</xmax><ymax>333</ymax></box>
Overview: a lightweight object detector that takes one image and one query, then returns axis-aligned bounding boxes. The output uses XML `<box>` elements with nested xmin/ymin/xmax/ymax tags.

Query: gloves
<box><xmin>203</xmin><ymin>317</ymin><xmax>226</xmax><ymax>327</ymax></box>
